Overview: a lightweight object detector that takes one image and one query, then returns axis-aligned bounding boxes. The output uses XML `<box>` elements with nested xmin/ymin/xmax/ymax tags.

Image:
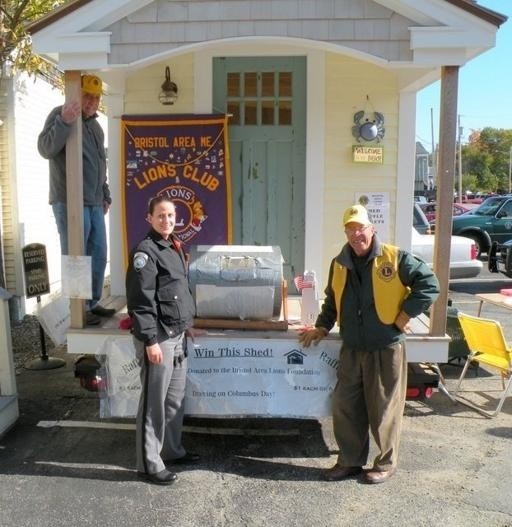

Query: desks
<box><xmin>474</xmin><ymin>293</ymin><xmax>512</xmax><ymax>318</ymax></box>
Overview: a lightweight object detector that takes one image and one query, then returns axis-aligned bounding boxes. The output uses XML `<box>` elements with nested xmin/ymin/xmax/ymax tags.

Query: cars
<box><xmin>488</xmin><ymin>237</ymin><xmax>512</xmax><ymax>279</ymax></box>
<box><xmin>418</xmin><ymin>203</ymin><xmax>470</xmax><ymax>222</ymax></box>
<box><xmin>413</xmin><ymin>203</ymin><xmax>431</xmax><ymax>236</ymax></box>
<box><xmin>407</xmin><ymin>220</ymin><xmax>483</xmax><ymax>283</ymax></box>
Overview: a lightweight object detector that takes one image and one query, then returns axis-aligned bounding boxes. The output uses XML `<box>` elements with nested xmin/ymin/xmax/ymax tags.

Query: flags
<box><xmin>117</xmin><ymin>113</ymin><xmax>234</xmax><ymax>285</ymax></box>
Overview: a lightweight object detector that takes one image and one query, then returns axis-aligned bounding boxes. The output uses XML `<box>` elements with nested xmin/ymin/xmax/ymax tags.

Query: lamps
<box><xmin>157</xmin><ymin>64</ymin><xmax>178</xmax><ymax>105</ymax></box>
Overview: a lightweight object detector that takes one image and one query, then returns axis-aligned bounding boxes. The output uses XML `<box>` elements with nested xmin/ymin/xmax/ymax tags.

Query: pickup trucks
<box><xmin>433</xmin><ymin>195</ymin><xmax>512</xmax><ymax>258</ymax></box>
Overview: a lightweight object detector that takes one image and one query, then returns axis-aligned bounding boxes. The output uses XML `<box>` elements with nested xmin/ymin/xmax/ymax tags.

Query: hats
<box><xmin>343</xmin><ymin>204</ymin><xmax>370</xmax><ymax>226</ymax></box>
<box><xmin>81</xmin><ymin>73</ymin><xmax>103</xmax><ymax>98</ymax></box>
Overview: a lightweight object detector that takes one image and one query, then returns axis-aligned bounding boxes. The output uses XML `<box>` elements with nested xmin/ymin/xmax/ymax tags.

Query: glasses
<box><xmin>344</xmin><ymin>225</ymin><xmax>370</xmax><ymax>235</ymax></box>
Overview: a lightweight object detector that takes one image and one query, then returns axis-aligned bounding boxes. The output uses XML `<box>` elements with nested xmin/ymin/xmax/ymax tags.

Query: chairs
<box><xmin>453</xmin><ymin>310</ymin><xmax>512</xmax><ymax>419</ymax></box>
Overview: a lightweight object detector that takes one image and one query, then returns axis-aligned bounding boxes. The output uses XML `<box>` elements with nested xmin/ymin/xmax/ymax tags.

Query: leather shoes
<box><xmin>92</xmin><ymin>304</ymin><xmax>116</xmax><ymax>317</ymax></box>
<box><xmin>85</xmin><ymin>310</ymin><xmax>102</xmax><ymax>326</ymax></box>
<box><xmin>324</xmin><ymin>464</ymin><xmax>362</xmax><ymax>481</ymax></box>
<box><xmin>365</xmin><ymin>467</ymin><xmax>395</xmax><ymax>484</ymax></box>
<box><xmin>137</xmin><ymin>468</ymin><xmax>178</xmax><ymax>484</ymax></box>
<box><xmin>163</xmin><ymin>453</ymin><xmax>201</xmax><ymax>464</ymax></box>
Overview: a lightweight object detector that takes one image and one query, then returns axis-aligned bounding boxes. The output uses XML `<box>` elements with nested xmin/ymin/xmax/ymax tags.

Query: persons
<box><xmin>37</xmin><ymin>75</ymin><xmax>116</xmax><ymax>328</ymax></box>
<box><xmin>298</xmin><ymin>205</ymin><xmax>442</xmax><ymax>485</ymax></box>
<box><xmin>125</xmin><ymin>196</ymin><xmax>208</xmax><ymax>485</ymax></box>
